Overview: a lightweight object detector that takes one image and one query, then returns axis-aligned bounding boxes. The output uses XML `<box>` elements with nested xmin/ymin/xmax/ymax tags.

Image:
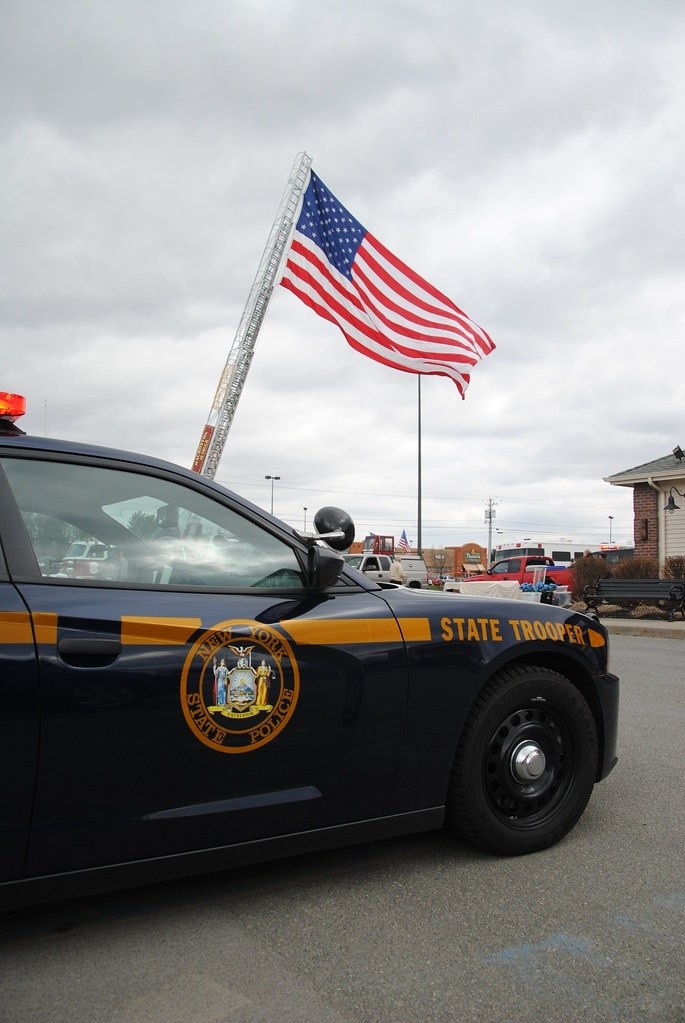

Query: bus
<box><xmin>494</xmin><ymin>540</ymin><xmax>634</xmax><ymax>569</ymax></box>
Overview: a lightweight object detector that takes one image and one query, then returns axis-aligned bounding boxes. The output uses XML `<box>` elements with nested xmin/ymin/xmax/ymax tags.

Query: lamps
<box><xmin>673</xmin><ymin>445</ymin><xmax>685</xmax><ymax>463</ymax></box>
<box><xmin>662</xmin><ymin>486</ymin><xmax>685</xmax><ymax>515</ymax></box>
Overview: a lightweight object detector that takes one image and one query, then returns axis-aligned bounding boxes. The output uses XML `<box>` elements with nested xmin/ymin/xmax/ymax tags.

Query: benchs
<box><xmin>581</xmin><ymin>573</ymin><xmax>685</xmax><ymax>622</ymax></box>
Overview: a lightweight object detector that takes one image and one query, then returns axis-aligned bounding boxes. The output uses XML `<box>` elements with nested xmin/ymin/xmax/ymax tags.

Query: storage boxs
<box><xmin>521</xmin><ymin>586</ymin><xmax>572</xmax><ymax>605</ymax></box>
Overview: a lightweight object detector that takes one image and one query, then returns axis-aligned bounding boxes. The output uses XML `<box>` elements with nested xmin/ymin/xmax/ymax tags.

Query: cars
<box><xmin>1</xmin><ymin>393</ymin><xmax>621</xmax><ymax>917</ymax></box>
<box><xmin>438</xmin><ymin>575</ymin><xmax>458</xmax><ymax>582</ymax></box>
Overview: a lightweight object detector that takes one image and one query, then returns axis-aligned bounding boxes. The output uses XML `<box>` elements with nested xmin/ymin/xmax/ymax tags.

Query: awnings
<box><xmin>462</xmin><ymin>564</ymin><xmax>485</xmax><ymax>571</ymax></box>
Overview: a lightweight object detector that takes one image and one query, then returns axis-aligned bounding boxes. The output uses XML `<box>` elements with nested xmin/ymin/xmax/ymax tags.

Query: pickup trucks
<box><xmin>340</xmin><ymin>554</ymin><xmax>429</xmax><ymax>591</ymax></box>
<box><xmin>463</xmin><ymin>555</ymin><xmax>575</xmax><ymax>592</ymax></box>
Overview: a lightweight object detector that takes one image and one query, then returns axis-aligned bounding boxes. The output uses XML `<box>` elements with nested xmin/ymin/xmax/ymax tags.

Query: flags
<box><xmin>280</xmin><ymin>168</ymin><xmax>496</xmax><ymax>400</ymax></box>
<box><xmin>398</xmin><ymin>531</ymin><xmax>411</xmax><ymax>556</ymax></box>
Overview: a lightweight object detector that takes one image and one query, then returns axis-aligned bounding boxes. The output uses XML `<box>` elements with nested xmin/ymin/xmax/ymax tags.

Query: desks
<box><xmin>445</xmin><ymin>581</ymin><xmax>521</xmax><ymax>599</ymax></box>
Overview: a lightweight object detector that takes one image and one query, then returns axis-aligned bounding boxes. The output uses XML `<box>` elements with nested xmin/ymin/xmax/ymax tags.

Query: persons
<box><xmin>390</xmin><ymin>556</ymin><xmax>407</xmax><ymax>585</ymax></box>
<box><xmin>584</xmin><ymin>549</ymin><xmax>613</xmax><ymax>580</ymax></box>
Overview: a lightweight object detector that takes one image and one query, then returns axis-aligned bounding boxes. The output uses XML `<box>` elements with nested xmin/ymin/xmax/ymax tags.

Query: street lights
<box><xmin>303</xmin><ymin>507</ymin><xmax>307</xmax><ymax>531</ymax></box>
<box><xmin>265</xmin><ymin>476</ymin><xmax>280</xmax><ymax>515</ymax></box>
<box><xmin>608</xmin><ymin>516</ymin><xmax>614</xmax><ymax>544</ymax></box>
